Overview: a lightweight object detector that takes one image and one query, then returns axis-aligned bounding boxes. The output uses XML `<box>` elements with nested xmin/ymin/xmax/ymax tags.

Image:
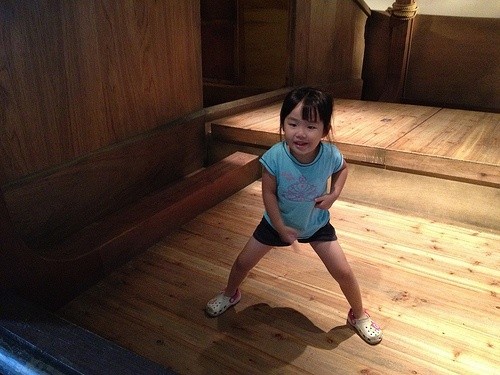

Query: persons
<box><xmin>205</xmin><ymin>84</ymin><xmax>383</xmax><ymax>345</ymax></box>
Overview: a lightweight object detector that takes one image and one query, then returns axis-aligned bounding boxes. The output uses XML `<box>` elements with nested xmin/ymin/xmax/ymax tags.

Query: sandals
<box><xmin>346</xmin><ymin>308</ymin><xmax>382</xmax><ymax>346</ymax></box>
<box><xmin>206</xmin><ymin>288</ymin><xmax>241</xmax><ymax>317</ymax></box>
